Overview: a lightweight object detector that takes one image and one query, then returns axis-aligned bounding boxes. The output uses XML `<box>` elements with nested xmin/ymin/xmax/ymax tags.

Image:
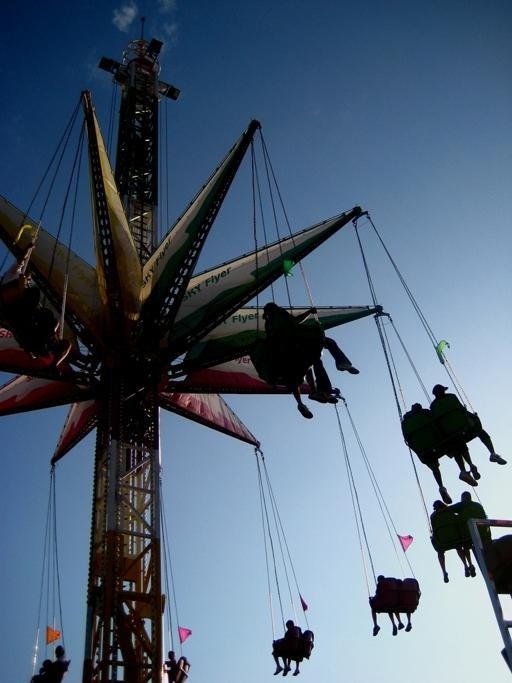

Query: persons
<box><xmin>261</xmin><ymin>301</ymin><xmax>359</xmax><ymax>419</ymax></box>
<box><xmin>29</xmin><ymin>644</ymin><xmax>67</xmax><ymax>683</ymax></box>
<box><xmin>272</xmin><ymin>619</ymin><xmax>315</xmax><ymax>678</ymax></box>
<box><xmin>429</xmin><ymin>490</ymin><xmax>494</xmax><ymax>583</ymax></box>
<box><xmin>371</xmin><ymin>576</ymin><xmax>422</xmax><ymax>637</ymax></box>
<box><xmin>163</xmin><ymin>651</ymin><xmax>190</xmax><ymax>683</ymax></box>
<box><xmin>1</xmin><ymin>239</ymin><xmax>64</xmax><ymax>355</ymax></box>
<box><xmin>401</xmin><ymin>384</ymin><xmax>507</xmax><ymax>504</ymax></box>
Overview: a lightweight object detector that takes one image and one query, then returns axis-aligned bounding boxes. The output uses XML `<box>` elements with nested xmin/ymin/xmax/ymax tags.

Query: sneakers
<box><xmin>393</xmin><ymin>623</ymin><xmax>411</xmax><ymax>635</ymax></box>
<box><xmin>465</xmin><ymin>565</ymin><xmax>476</xmax><ymax>577</ymax></box>
<box><xmin>309</xmin><ymin>391</ymin><xmax>338</xmax><ymax>403</ymax></box>
<box><xmin>373</xmin><ymin>625</ymin><xmax>380</xmax><ymax>636</ymax></box>
<box><xmin>337</xmin><ymin>363</ymin><xmax>359</xmax><ymax>374</ymax></box>
<box><xmin>459</xmin><ymin>464</ymin><xmax>480</xmax><ymax>486</ymax></box>
<box><xmin>54</xmin><ymin>339</ymin><xmax>72</xmax><ymax>368</ymax></box>
<box><xmin>444</xmin><ymin>573</ymin><xmax>449</xmax><ymax>583</ymax></box>
<box><xmin>490</xmin><ymin>454</ymin><xmax>506</xmax><ymax>465</ymax></box>
<box><xmin>439</xmin><ymin>487</ymin><xmax>452</xmax><ymax>504</ymax></box>
<box><xmin>298</xmin><ymin>404</ymin><xmax>313</xmax><ymax>419</ymax></box>
<box><xmin>274</xmin><ymin>667</ymin><xmax>300</xmax><ymax>676</ymax></box>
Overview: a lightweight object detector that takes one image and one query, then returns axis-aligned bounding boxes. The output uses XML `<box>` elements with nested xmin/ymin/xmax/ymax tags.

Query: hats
<box><xmin>433</xmin><ymin>500</ymin><xmax>443</xmax><ymax>509</ymax></box>
<box><xmin>433</xmin><ymin>385</ymin><xmax>448</xmax><ymax>393</ymax></box>
